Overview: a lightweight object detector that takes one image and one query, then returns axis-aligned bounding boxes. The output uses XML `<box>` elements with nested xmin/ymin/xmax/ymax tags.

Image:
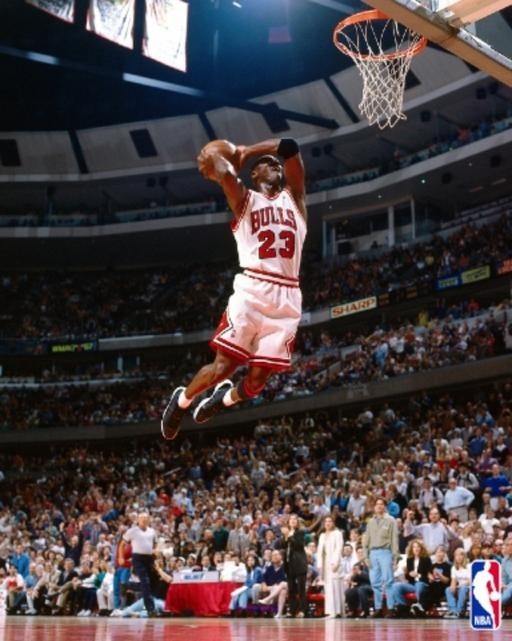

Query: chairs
<box><xmin>292</xmin><ymin>592</ymin><xmax>418</xmax><ymax>617</ymax></box>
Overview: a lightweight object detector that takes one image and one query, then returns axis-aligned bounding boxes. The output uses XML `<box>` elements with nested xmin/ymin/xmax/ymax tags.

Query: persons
<box><xmin>0</xmin><ymin>115</ymin><xmax>512</xmax><ymax>620</ymax></box>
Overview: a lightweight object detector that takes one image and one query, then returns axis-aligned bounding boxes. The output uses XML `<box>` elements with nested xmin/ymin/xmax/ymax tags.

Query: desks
<box><xmin>164</xmin><ymin>582</ymin><xmax>242</xmax><ymax>617</ymax></box>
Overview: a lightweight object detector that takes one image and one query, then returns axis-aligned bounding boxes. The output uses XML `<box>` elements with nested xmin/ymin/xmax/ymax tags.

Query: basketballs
<box><xmin>198</xmin><ymin>139</ymin><xmax>241</xmax><ymax>181</ymax></box>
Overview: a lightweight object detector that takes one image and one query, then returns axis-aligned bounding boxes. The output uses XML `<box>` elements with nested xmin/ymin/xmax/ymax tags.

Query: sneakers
<box><xmin>193</xmin><ymin>379</ymin><xmax>232</xmax><ymax>424</ymax></box>
<box><xmin>444</xmin><ymin>610</ymin><xmax>460</xmax><ymax>618</ymax></box>
<box><xmin>370</xmin><ymin>604</ymin><xmax>426</xmax><ymax>618</ymax></box>
<box><xmin>161</xmin><ymin>387</ymin><xmax>193</xmax><ymax>439</ymax></box>
<box><xmin>6</xmin><ymin>605</ymin><xmax>112</xmax><ymax>617</ymax></box>
<box><xmin>230</xmin><ymin>601</ymin><xmax>285</xmax><ymax>619</ymax></box>
